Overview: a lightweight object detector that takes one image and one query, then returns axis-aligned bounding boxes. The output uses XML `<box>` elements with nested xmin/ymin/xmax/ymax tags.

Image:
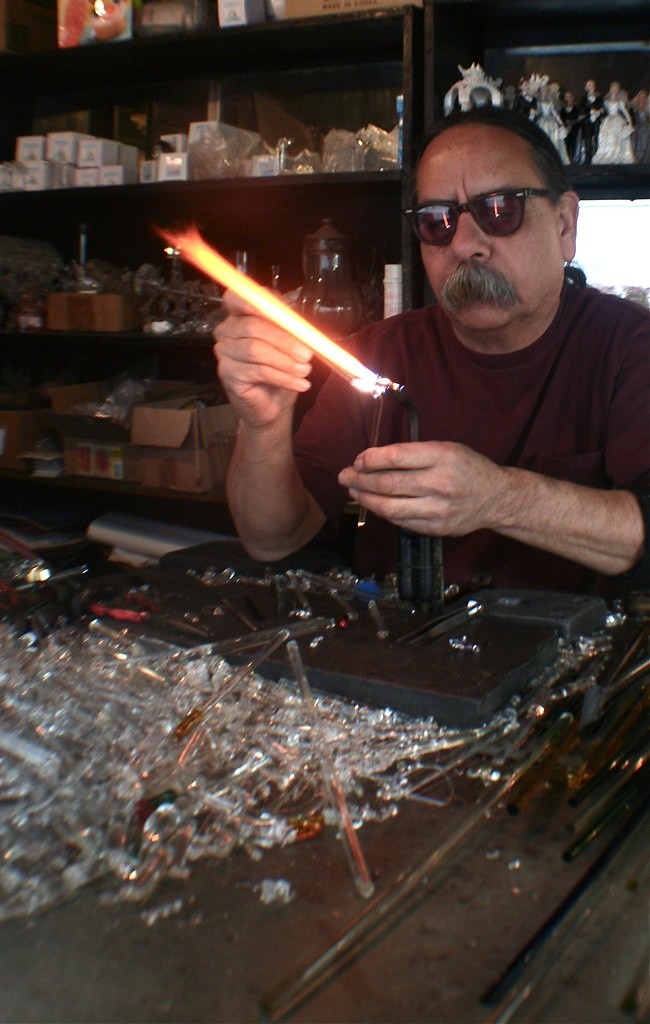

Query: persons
<box><xmin>210</xmin><ymin>109</ymin><xmax>650</xmax><ymax>594</ymax></box>
<box><xmin>512</xmin><ymin>75</ymin><xmax>650</xmax><ymax>166</ymax></box>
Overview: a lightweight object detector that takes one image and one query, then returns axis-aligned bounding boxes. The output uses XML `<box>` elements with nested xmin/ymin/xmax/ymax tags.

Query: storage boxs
<box><xmin>218</xmin><ymin>1</ymin><xmax>266</xmax><ymax>27</ymax></box>
<box><xmin>128</xmin><ymin>386</ymin><xmax>239</xmax><ymax>493</ymax></box>
<box><xmin>90</xmin><ymin>293</ymin><xmax>147</xmax><ymax>332</ymax></box>
<box><xmin>141</xmin><ymin>0</ymin><xmax>214</xmax><ymax>38</ymax></box>
<box><xmin>48</xmin><ymin>375</ymin><xmax>222</xmax><ymax>484</ymax></box>
<box><xmin>285</xmin><ymin>0</ymin><xmax>424</xmax><ymax>19</ymax></box>
<box><xmin>46</xmin><ymin>292</ymin><xmax>93</xmax><ymax>331</ymax></box>
<box><xmin>56</xmin><ymin>0</ymin><xmax>145</xmax><ymax>48</ymax></box>
<box><xmin>0</xmin><ymin>384</ymin><xmax>62</xmax><ymax>472</ymax></box>
<box><xmin>0</xmin><ymin>0</ymin><xmax>58</xmax><ymax>59</ymax></box>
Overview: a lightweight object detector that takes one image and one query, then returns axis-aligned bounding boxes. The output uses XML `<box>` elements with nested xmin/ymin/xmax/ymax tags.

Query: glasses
<box><xmin>404</xmin><ymin>186</ymin><xmax>549</xmax><ymax>246</ymax></box>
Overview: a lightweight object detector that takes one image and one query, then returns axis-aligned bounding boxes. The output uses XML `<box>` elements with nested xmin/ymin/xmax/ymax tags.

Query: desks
<box><xmin>0</xmin><ymin>539</ymin><xmax>649</xmax><ymax>1024</ymax></box>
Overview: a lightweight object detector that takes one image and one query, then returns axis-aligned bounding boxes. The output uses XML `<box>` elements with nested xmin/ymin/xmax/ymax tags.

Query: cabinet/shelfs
<box><xmin>0</xmin><ymin>0</ymin><xmax>424</xmax><ymax>538</ymax></box>
<box><xmin>422</xmin><ymin>0</ymin><xmax>649</xmax><ymax>307</ymax></box>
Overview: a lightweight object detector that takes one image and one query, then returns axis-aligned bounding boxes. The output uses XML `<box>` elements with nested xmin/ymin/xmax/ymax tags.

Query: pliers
<box><xmin>86</xmin><ymin>586</ymin><xmax>215</xmax><ymax>640</ymax></box>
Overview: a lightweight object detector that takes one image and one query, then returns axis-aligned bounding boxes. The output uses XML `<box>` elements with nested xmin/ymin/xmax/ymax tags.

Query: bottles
<box><xmin>295</xmin><ymin>218</ymin><xmax>378</xmax><ymax>336</ymax></box>
